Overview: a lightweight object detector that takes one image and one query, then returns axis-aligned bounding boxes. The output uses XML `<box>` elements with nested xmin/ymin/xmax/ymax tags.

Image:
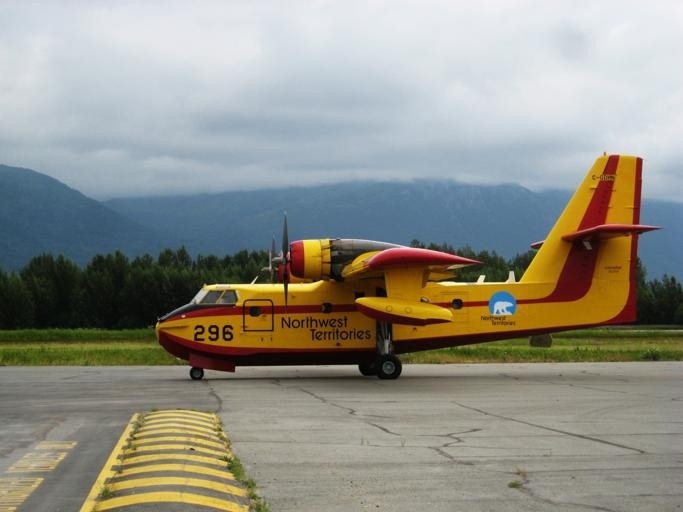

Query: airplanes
<box><xmin>151</xmin><ymin>151</ymin><xmax>659</xmax><ymax>385</ymax></box>
